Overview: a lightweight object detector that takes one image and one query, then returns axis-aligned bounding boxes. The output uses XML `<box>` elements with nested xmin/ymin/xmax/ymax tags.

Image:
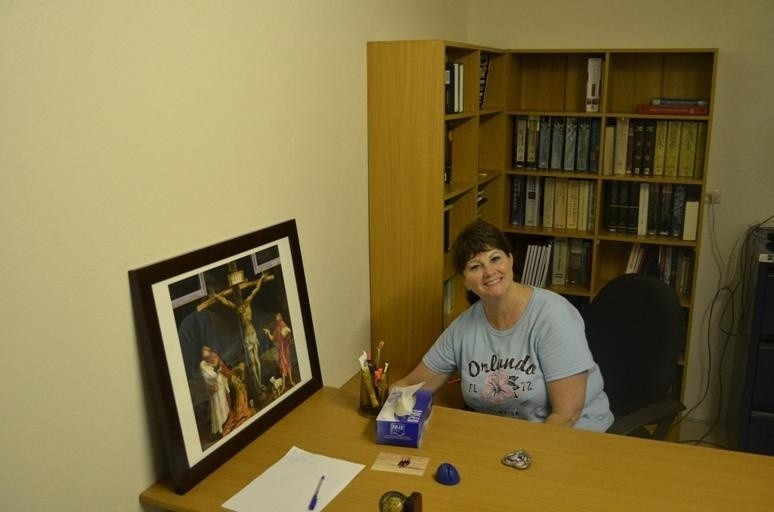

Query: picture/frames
<box><xmin>126</xmin><ymin>217</ymin><xmax>324</xmax><ymax>496</ymax></box>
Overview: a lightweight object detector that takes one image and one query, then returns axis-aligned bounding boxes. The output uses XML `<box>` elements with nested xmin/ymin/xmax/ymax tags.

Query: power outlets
<box><xmin>704</xmin><ymin>188</ymin><xmax>721</xmax><ymax>205</ymax></box>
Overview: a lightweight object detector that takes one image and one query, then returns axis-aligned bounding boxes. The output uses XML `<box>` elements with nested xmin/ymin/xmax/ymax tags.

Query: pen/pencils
<box><xmin>358</xmin><ymin>340</ymin><xmax>389</xmax><ymax>412</ymax></box>
<box><xmin>308</xmin><ymin>475</ymin><xmax>324</xmax><ymax>510</ymax></box>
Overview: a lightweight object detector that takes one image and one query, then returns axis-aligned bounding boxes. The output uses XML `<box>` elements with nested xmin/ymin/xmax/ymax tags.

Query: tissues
<box><xmin>375</xmin><ymin>381</ymin><xmax>434</xmax><ymax>448</ymax></box>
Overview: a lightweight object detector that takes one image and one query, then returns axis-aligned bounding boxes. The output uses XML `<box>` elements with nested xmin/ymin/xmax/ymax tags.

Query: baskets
<box><xmin>358</xmin><ymin>369</ymin><xmax>389</xmax><ymax>418</ymax></box>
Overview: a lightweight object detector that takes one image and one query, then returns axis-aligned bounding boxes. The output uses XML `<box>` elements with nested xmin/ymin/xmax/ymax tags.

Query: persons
<box><xmin>201</xmin><ymin>273</ymin><xmax>297</xmax><ymax>434</ymax></box>
<box><xmin>384</xmin><ymin>221</ymin><xmax>615</xmax><ymax>434</ymax></box>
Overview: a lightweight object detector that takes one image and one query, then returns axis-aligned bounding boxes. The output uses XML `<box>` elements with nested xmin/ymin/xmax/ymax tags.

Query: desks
<box><xmin>137</xmin><ymin>384</ymin><xmax>773</xmax><ymax>512</ymax></box>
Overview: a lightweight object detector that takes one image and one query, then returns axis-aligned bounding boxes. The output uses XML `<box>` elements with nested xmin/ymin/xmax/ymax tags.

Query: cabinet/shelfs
<box><xmin>365</xmin><ymin>40</ymin><xmax>509</xmax><ymax>409</ymax></box>
<box><xmin>502</xmin><ymin>47</ymin><xmax>720</xmax><ymax>442</ymax></box>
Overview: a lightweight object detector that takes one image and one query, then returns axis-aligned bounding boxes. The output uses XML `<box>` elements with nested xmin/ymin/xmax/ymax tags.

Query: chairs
<box><xmin>583</xmin><ymin>272</ymin><xmax>688</xmax><ymax>441</ymax></box>
